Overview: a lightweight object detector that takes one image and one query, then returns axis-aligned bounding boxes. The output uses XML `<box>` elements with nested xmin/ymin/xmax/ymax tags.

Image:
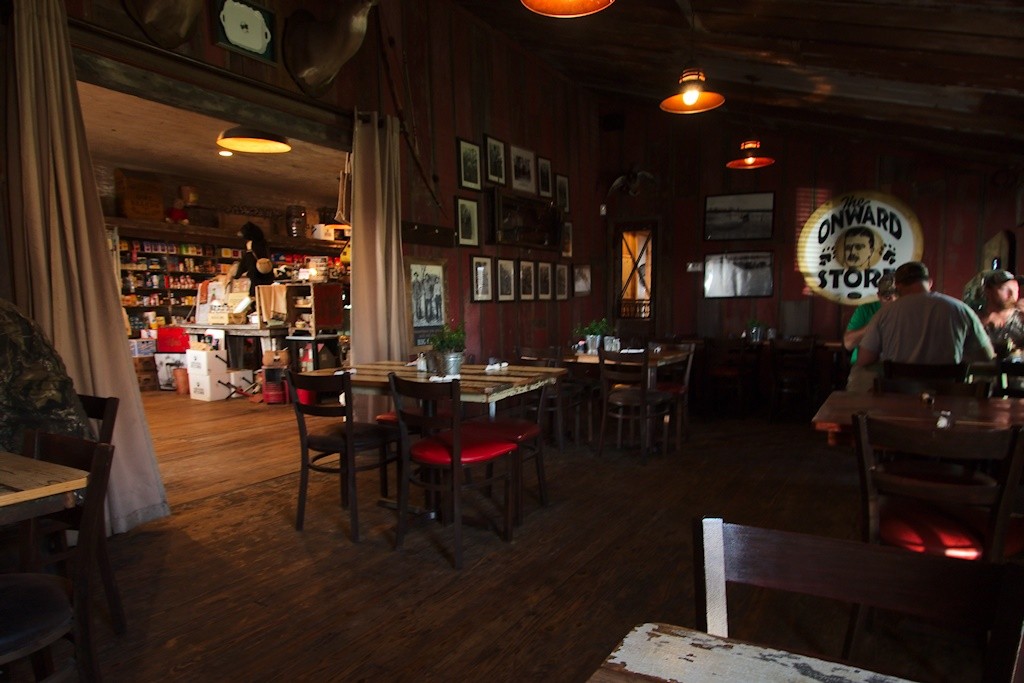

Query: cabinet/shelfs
<box><xmin>285</xmin><ymin>281</ymin><xmax>344</xmax><ymax>337</ymax></box>
<box><xmin>110</xmin><ymin>226</ymin><xmax>341</xmax><ymax>339</ymax></box>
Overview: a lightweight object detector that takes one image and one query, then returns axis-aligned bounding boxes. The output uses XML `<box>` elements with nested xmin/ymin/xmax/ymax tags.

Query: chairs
<box><xmin>517</xmin><ymin>336</ymin><xmax>1024</xmax><ymax>683</ymax></box>
<box><xmin>285</xmin><ymin>370</ymin><xmax>554</xmax><ymax>569</ymax></box>
<box><xmin>0</xmin><ymin>396</ymin><xmax>126</xmax><ymax>683</ymax></box>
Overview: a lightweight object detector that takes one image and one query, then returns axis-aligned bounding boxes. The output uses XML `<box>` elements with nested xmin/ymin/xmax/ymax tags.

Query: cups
<box><xmin>604</xmin><ymin>336</ymin><xmax>615</xmax><ymax>351</ymax></box>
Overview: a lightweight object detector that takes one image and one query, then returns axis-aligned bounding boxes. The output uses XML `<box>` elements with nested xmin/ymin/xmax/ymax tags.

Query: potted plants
<box><xmin>572</xmin><ymin>316</ymin><xmax>610</xmax><ymax>355</ymax></box>
<box><xmin>424</xmin><ymin>319</ymin><xmax>467</xmax><ymax>374</ymax></box>
<box><xmin>747</xmin><ymin>318</ymin><xmax>764</xmax><ymax>344</ymax></box>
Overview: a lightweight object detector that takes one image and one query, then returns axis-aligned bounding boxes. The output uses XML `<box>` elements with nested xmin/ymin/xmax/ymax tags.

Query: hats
<box><xmin>986</xmin><ymin>271</ymin><xmax>1024</xmax><ymax>288</ymax></box>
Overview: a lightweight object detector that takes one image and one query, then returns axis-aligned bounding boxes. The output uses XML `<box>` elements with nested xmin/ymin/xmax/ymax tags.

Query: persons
<box><xmin>858</xmin><ymin>260</ymin><xmax>994</xmax><ymax>464</ymax></box>
<box><xmin>0</xmin><ymin>298</ymin><xmax>95</xmax><ymax>531</ymax></box>
<box><xmin>844</xmin><ymin>270</ymin><xmax>896</xmax><ymax>388</ymax></box>
<box><xmin>962</xmin><ymin>269</ymin><xmax>1024</xmax><ymax>391</ymax></box>
<box><xmin>413</xmin><ymin>143</ymin><xmax>588</xmax><ymax>325</ymax></box>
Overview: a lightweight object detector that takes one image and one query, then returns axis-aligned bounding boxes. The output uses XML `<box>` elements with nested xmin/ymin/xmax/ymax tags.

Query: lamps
<box><xmin>657</xmin><ymin>0</ymin><xmax>725</xmax><ymax>114</ymax></box>
<box><xmin>724</xmin><ymin>76</ymin><xmax>778</xmax><ymax>169</ymax></box>
<box><xmin>521</xmin><ymin>0</ymin><xmax>617</xmax><ymax>19</ymax></box>
<box><xmin>215</xmin><ymin>124</ymin><xmax>292</xmax><ymax>153</ymax></box>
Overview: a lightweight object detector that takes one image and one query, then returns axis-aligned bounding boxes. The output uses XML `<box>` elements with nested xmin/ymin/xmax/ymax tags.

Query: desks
<box><xmin>285</xmin><ymin>333</ymin><xmax>339</xmax><ymax>402</ymax></box>
<box><xmin>812</xmin><ymin>391</ymin><xmax>1024</xmax><ymax>453</ymax></box>
<box><xmin>583</xmin><ymin>622</ymin><xmax>914</xmax><ymax>683</ymax></box>
<box><xmin>182</xmin><ymin>325</ymin><xmax>289</xmax><ymax>370</ymax></box>
<box><xmin>727</xmin><ymin>340</ymin><xmax>845</xmax><ymax>417</ymax></box>
<box><xmin>518</xmin><ymin>349</ymin><xmax>693</xmax><ymax>449</ymax></box>
<box><xmin>298</xmin><ymin>361</ymin><xmax>568</xmax><ymax>519</ymax></box>
<box><xmin>0</xmin><ymin>447</ymin><xmax>93</xmax><ymax>525</ymax></box>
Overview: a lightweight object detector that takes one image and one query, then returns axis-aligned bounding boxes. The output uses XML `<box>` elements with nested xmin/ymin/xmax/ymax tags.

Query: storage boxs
<box><xmin>312</xmin><ymin>224</ymin><xmax>334</xmax><ymax>240</ymax></box>
<box><xmin>128</xmin><ymin>312</ymin><xmax>337</xmax><ymax>401</ymax></box>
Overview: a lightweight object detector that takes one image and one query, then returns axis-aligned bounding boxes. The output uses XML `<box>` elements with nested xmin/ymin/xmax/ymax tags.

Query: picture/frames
<box><xmin>455</xmin><ymin>133</ymin><xmax>593</xmax><ymax>302</ymax></box>
<box><xmin>403</xmin><ymin>254</ymin><xmax>448</xmax><ymax>356</ymax></box>
<box><xmin>702</xmin><ymin>191</ymin><xmax>774</xmax><ymax>299</ymax></box>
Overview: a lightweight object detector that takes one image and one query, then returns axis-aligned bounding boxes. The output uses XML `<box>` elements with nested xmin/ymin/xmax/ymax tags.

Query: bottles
<box><xmin>612</xmin><ymin>338</ymin><xmax>620</xmax><ymax>352</ymax></box>
<box><xmin>126</xmin><ymin>258</ymin><xmax>199</xmax><ymax>330</ymax></box>
<box><xmin>416</xmin><ymin>350</ymin><xmax>438</xmax><ymax>372</ymax></box>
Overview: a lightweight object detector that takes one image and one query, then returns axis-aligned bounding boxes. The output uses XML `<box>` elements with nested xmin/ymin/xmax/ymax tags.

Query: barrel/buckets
<box><xmin>286</xmin><ymin>205</ymin><xmax>307</xmax><ymax>237</ymax></box>
